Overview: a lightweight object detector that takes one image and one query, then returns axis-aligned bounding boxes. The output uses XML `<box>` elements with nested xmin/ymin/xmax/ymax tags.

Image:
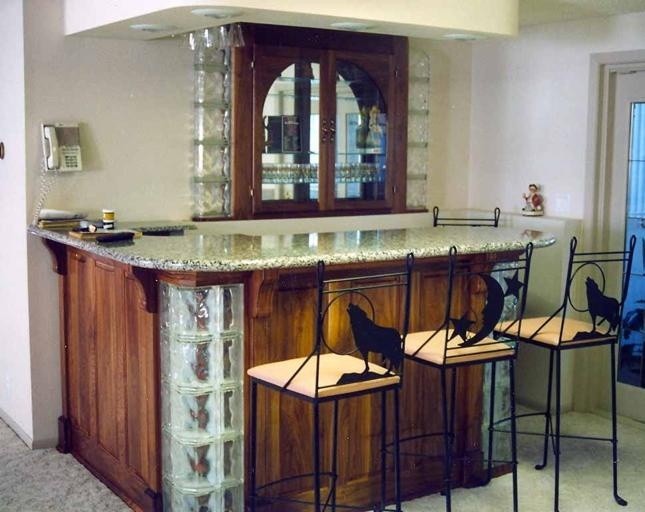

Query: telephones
<box><xmin>40</xmin><ymin>122</ymin><xmax>82</xmax><ymax>172</ymax></box>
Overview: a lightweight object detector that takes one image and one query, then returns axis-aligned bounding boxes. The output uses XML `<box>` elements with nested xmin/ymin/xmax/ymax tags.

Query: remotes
<box><xmin>97</xmin><ymin>232</ymin><xmax>133</xmax><ymax>242</ymax></box>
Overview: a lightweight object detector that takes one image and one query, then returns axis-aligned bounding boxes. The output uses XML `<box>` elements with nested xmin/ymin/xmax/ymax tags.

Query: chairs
<box><xmin>379</xmin><ymin>241</ymin><xmax>534</xmax><ymax>509</ymax></box>
<box><xmin>433</xmin><ymin>206</ymin><xmax>500</xmax><ymax>227</ymax></box>
<box><xmin>246</xmin><ymin>253</ymin><xmax>415</xmax><ymax>510</ymax></box>
<box><xmin>482</xmin><ymin>233</ymin><xmax>637</xmax><ymax>510</ymax></box>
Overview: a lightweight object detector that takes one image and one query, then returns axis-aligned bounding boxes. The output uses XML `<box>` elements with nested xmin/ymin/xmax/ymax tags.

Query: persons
<box><xmin>522</xmin><ymin>184</ymin><xmax>542</xmax><ymax>211</ymax></box>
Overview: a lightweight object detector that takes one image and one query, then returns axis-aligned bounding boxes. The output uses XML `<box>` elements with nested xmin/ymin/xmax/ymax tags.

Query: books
<box><xmin>37</xmin><ymin>209</ymin><xmax>142</xmax><ymax>241</ymax></box>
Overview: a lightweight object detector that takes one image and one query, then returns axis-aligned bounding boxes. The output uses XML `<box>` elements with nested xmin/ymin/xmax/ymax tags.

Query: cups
<box><xmin>259</xmin><ymin>163</ymin><xmax>386</xmax><ymax>183</ymax></box>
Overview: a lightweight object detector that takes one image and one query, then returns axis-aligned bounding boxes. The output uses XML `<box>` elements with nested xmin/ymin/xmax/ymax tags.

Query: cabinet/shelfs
<box><xmin>228</xmin><ymin>26</ymin><xmax>408</xmax><ymax>219</ymax></box>
